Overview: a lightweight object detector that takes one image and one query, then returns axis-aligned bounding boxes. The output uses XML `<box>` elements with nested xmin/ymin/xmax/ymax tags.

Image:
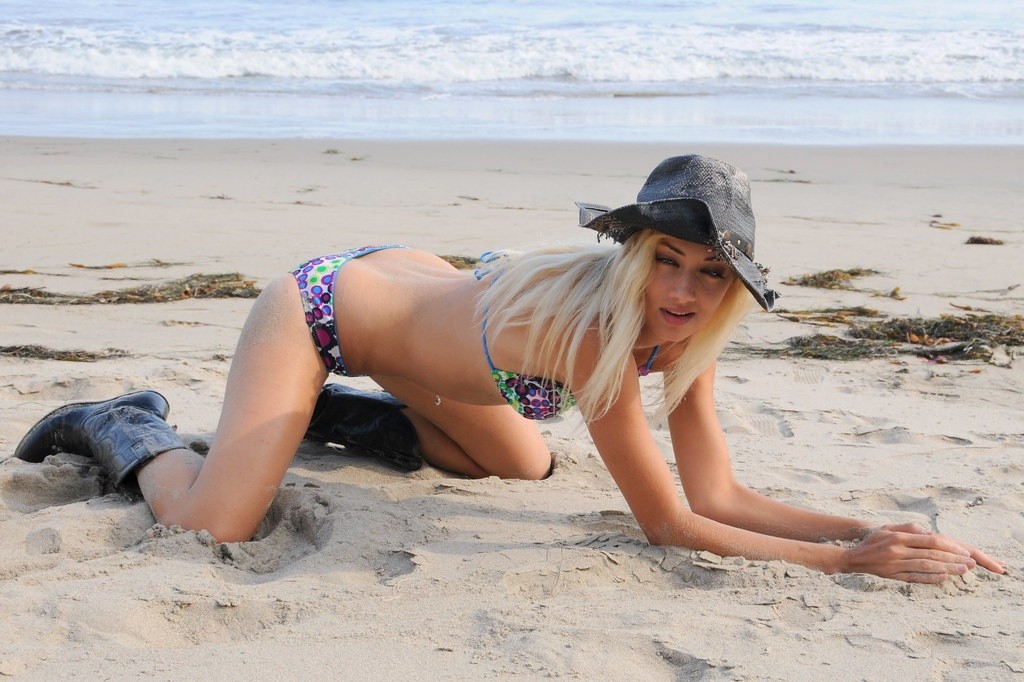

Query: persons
<box><xmin>14</xmin><ymin>155</ymin><xmax>1004</xmax><ymax>585</ymax></box>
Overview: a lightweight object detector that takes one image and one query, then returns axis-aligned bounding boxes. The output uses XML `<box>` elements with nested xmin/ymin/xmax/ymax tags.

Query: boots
<box><xmin>15</xmin><ymin>391</ymin><xmax>187</xmax><ymax>498</ymax></box>
<box><xmin>305</xmin><ymin>383</ymin><xmax>422</xmax><ymax>470</ymax></box>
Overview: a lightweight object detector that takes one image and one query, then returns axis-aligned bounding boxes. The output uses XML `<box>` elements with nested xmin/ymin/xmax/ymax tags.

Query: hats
<box><xmin>574</xmin><ymin>153</ymin><xmax>782</xmax><ymax>313</ymax></box>
